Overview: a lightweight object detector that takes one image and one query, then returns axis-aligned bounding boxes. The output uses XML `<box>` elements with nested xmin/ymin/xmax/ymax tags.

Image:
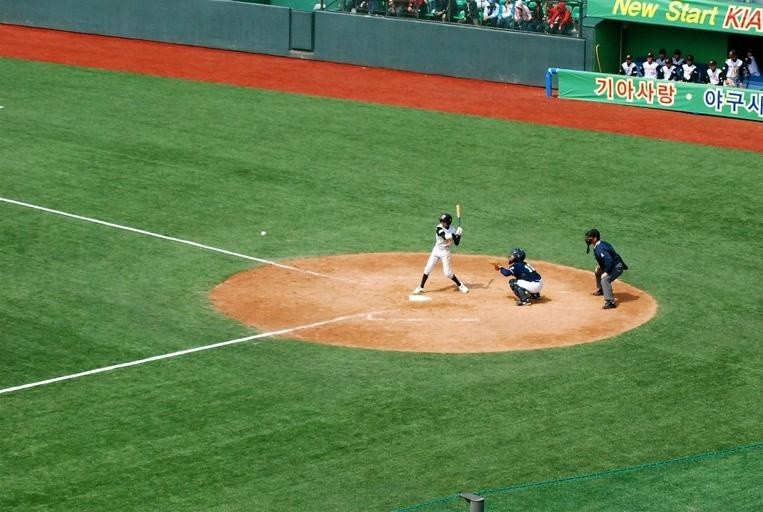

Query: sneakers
<box><xmin>413</xmin><ymin>287</ymin><xmax>426</xmax><ymax>294</ymax></box>
<box><xmin>604</xmin><ymin>300</ymin><xmax>616</xmax><ymax>308</ymax></box>
<box><xmin>593</xmin><ymin>288</ymin><xmax>603</xmax><ymax>296</ymax></box>
<box><xmin>457</xmin><ymin>282</ymin><xmax>469</xmax><ymax>293</ymax></box>
<box><xmin>528</xmin><ymin>293</ymin><xmax>540</xmax><ymax>299</ymax></box>
<box><xmin>517</xmin><ymin>298</ymin><xmax>531</xmax><ymax>305</ymax></box>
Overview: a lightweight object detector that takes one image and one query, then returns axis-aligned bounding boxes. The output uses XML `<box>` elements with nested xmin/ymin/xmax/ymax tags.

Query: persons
<box><xmin>499</xmin><ymin>248</ymin><xmax>544</xmax><ymax>306</ymax></box>
<box><xmin>584</xmin><ymin>229</ymin><xmax>623</xmax><ymax>309</ymax></box>
<box><xmin>619</xmin><ymin>48</ymin><xmax>760</xmax><ymax>88</ymax></box>
<box><xmin>367</xmin><ymin>0</ymin><xmax>573</xmax><ymax>35</ymax></box>
<box><xmin>414</xmin><ymin>213</ymin><xmax>468</xmax><ymax>295</ymax></box>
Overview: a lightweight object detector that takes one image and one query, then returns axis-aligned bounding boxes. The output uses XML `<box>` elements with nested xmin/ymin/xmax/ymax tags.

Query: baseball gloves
<box><xmin>489</xmin><ymin>257</ymin><xmax>502</xmax><ymax>271</ymax></box>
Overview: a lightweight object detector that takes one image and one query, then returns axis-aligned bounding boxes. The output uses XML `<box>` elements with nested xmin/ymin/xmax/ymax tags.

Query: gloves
<box><xmin>456</xmin><ymin>227</ymin><xmax>463</xmax><ymax>236</ymax></box>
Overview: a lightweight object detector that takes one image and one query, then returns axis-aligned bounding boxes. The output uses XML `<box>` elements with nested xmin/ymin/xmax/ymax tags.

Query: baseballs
<box><xmin>456</xmin><ymin>204</ymin><xmax>461</xmax><ymax>226</ymax></box>
<box><xmin>260</xmin><ymin>231</ymin><xmax>266</xmax><ymax>236</ymax></box>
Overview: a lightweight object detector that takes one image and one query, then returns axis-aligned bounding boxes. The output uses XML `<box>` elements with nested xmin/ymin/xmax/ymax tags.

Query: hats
<box><xmin>626</xmin><ymin>49</ymin><xmax>737</xmax><ymax>66</ymax></box>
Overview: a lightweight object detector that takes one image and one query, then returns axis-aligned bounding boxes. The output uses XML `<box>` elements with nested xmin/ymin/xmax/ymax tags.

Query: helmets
<box><xmin>585</xmin><ymin>229</ymin><xmax>600</xmax><ymax>254</ymax></box>
<box><xmin>439</xmin><ymin>214</ymin><xmax>452</xmax><ymax>225</ymax></box>
<box><xmin>509</xmin><ymin>248</ymin><xmax>525</xmax><ymax>264</ymax></box>
